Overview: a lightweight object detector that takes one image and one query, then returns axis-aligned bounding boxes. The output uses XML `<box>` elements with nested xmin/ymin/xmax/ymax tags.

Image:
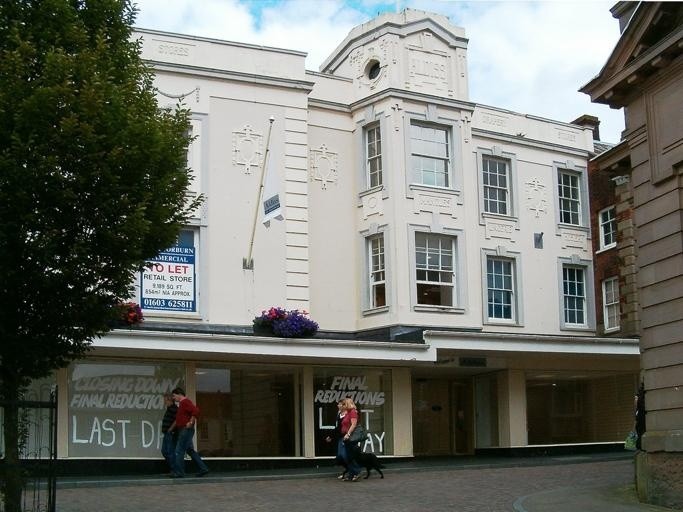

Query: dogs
<box><xmin>332</xmin><ymin>453</ymin><xmax>386</xmax><ymax>479</ymax></box>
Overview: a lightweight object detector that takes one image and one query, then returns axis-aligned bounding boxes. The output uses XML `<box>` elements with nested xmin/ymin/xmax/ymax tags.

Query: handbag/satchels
<box><xmin>349</xmin><ymin>425</ymin><xmax>369</xmax><ymax>445</ymax></box>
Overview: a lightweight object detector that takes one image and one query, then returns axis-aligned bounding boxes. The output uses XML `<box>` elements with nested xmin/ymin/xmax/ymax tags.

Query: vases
<box><xmin>253</xmin><ymin>317</ymin><xmax>273</xmax><ymax>337</ymax></box>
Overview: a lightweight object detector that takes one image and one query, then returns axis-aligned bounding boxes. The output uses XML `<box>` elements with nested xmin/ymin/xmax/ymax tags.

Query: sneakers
<box><xmin>195</xmin><ymin>468</ymin><xmax>209</xmax><ymax>477</ymax></box>
<box><xmin>338</xmin><ymin>473</ymin><xmax>363</xmax><ymax>482</ymax></box>
<box><xmin>168</xmin><ymin>472</ymin><xmax>185</xmax><ymax>478</ymax></box>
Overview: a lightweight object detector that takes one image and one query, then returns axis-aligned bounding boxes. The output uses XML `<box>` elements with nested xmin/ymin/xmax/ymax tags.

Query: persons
<box><xmin>323</xmin><ymin>397</ymin><xmax>361</xmax><ymax>481</ymax></box>
<box><xmin>632</xmin><ymin>393</ymin><xmax>639</xmax><ymax>463</ymax></box>
<box><xmin>168</xmin><ymin>387</ymin><xmax>210</xmax><ymax>478</ymax></box>
<box><xmin>161</xmin><ymin>392</ymin><xmax>179</xmax><ymax>477</ymax></box>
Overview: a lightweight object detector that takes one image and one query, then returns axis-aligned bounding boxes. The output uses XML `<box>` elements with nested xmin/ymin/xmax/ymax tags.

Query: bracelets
<box><xmin>189</xmin><ymin>419</ymin><xmax>195</xmax><ymax>424</ymax></box>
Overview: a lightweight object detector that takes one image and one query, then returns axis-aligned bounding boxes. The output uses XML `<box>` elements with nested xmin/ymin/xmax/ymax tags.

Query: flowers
<box><xmin>255</xmin><ymin>307</ymin><xmax>320</xmax><ymax>338</ymax></box>
<box><xmin>113</xmin><ymin>302</ymin><xmax>143</xmax><ymax>326</ymax></box>
